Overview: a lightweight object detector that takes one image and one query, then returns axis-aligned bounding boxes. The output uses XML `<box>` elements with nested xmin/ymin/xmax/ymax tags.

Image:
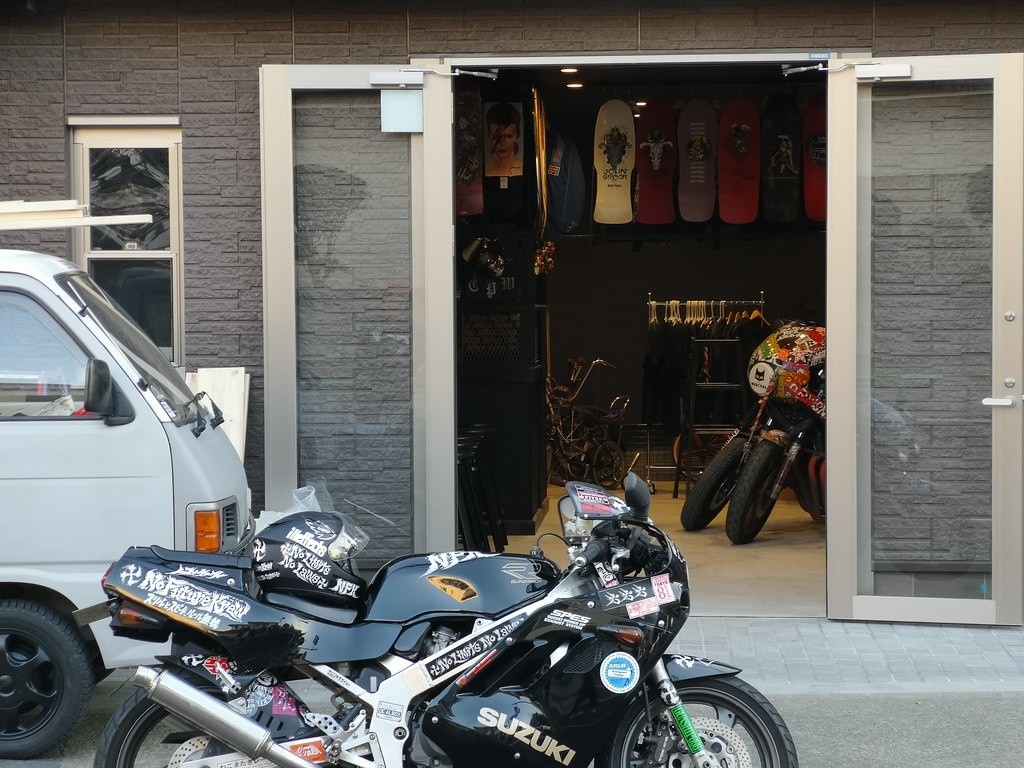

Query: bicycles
<box><xmin>542</xmin><ymin>358</ymin><xmax>635</xmax><ymax>492</ymax></box>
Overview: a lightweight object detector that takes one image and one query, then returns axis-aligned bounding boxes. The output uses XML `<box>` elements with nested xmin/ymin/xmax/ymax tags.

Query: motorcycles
<box><xmin>92</xmin><ymin>472</ymin><xmax>799</xmax><ymax>768</ymax></box>
<box><xmin>679</xmin><ymin>319</ymin><xmax>825</xmax><ymax>546</ymax></box>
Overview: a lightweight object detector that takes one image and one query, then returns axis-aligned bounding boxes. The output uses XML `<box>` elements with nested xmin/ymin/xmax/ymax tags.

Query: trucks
<box><xmin>1</xmin><ymin>250</ymin><xmax>254</xmax><ymax>763</ymax></box>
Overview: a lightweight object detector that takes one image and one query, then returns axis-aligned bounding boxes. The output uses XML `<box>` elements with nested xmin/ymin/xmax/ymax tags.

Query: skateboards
<box><xmin>587</xmin><ymin>91</ymin><xmax>829</xmax><ymax>225</ymax></box>
<box><xmin>527</xmin><ymin>81</ymin><xmax>557</xmax><ymax>246</ymax></box>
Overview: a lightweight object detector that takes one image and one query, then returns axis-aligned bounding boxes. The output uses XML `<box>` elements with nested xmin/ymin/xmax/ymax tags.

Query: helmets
<box><xmin>252</xmin><ymin>511</ymin><xmax>371</xmax><ymax>612</ymax></box>
